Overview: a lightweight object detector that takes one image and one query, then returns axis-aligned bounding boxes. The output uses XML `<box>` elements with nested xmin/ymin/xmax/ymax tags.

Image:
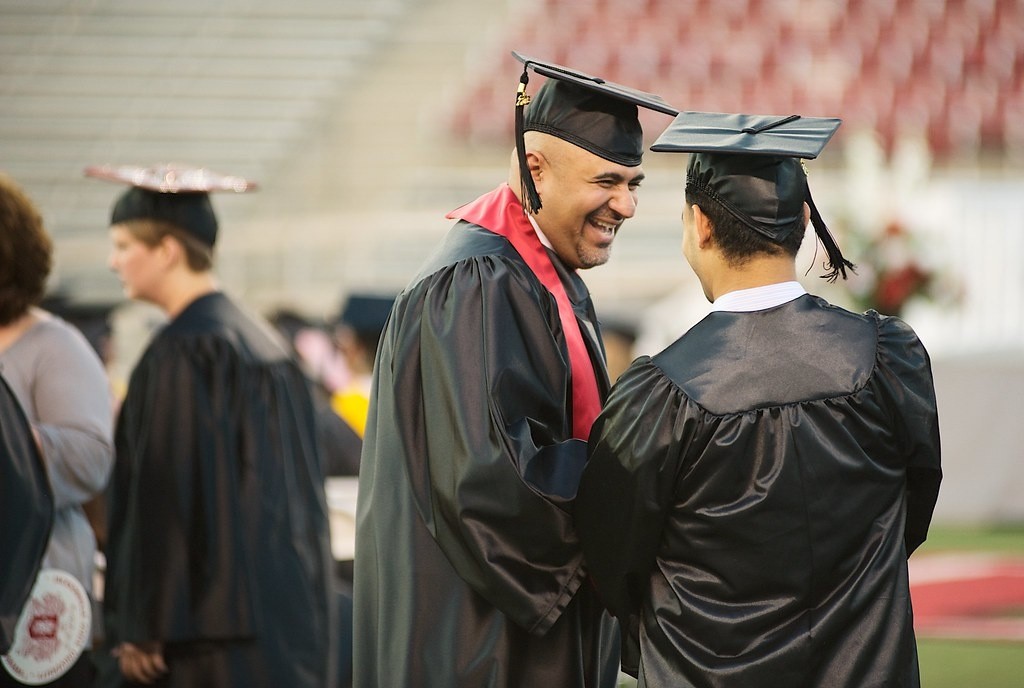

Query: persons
<box><xmin>350</xmin><ymin>57</ymin><xmax>680</xmax><ymax>637</ymax></box>
<box><xmin>571</xmin><ymin>110</ymin><xmax>940</xmax><ymax>688</ymax></box>
<box><xmin>0</xmin><ymin>172</ymin><xmax>108</xmax><ymax>685</ymax></box>
<box><xmin>85</xmin><ymin>163</ymin><xmax>343</xmax><ymax>687</ymax></box>
<box><xmin>45</xmin><ymin>289</ymin><xmax>644</xmax><ymax>612</ymax></box>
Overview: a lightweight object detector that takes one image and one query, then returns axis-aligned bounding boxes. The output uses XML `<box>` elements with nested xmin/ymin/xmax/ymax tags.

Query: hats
<box><xmin>649</xmin><ymin>109</ymin><xmax>860</xmax><ymax>281</ymax></box>
<box><xmin>510</xmin><ymin>50</ymin><xmax>681</xmax><ymax>214</ymax></box>
<box><xmin>83</xmin><ymin>160</ymin><xmax>261</xmax><ymax>244</ymax></box>
